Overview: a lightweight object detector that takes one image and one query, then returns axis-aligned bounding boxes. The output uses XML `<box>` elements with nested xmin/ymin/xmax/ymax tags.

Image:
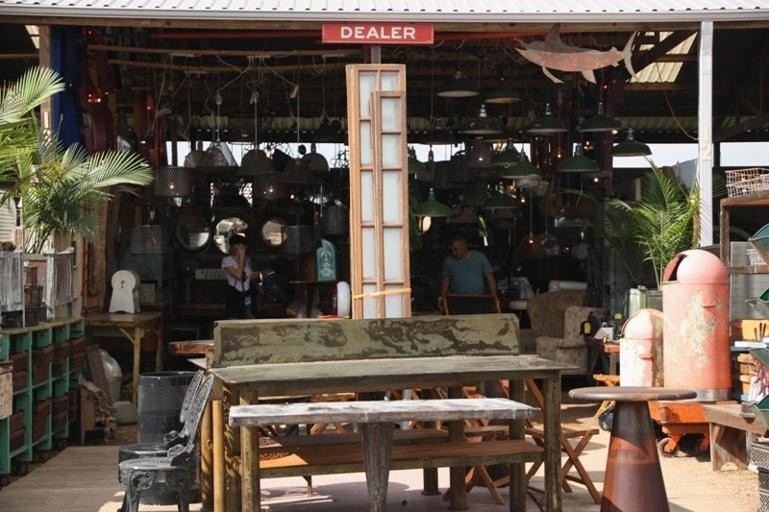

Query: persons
<box><xmin>440</xmin><ymin>234</ymin><xmax>497</xmax><ymax>300</ymax></box>
<box><xmin>221</xmin><ymin>234</ymin><xmax>259</xmax><ymax>318</ymax></box>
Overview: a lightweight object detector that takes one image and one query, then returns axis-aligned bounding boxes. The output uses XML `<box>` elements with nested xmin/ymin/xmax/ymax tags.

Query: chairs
<box><xmin>497</xmin><ymin>379</ymin><xmax>605</xmax><ymax>508</ymax></box>
<box><xmin>114</xmin><ymin>367</ymin><xmax>217</xmax><ymax>512</ymax></box>
<box><xmin>516</xmin><ymin>289</ymin><xmax>608</xmax><ymax>392</ymax></box>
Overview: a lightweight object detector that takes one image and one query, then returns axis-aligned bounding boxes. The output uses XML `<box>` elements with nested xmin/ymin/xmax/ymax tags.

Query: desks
<box><xmin>140</xmin><ymin>302</ymin><xmax>171</xmax><ymax>324</ymax></box>
<box><xmin>568</xmin><ymin>385</ymin><xmax>697</xmax><ymax>512</ymax></box>
<box><xmin>82</xmin><ymin>312</ymin><xmax>164</xmax><ymax>410</ymax></box>
<box><xmin>176</xmin><ymin>300</ymin><xmax>289</xmax><ymax>318</ymax></box>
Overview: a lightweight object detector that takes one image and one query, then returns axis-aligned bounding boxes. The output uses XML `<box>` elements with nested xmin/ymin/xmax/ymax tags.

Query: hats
<box><xmin>229</xmin><ymin>235</ymin><xmax>249</xmax><ymax>245</ymax></box>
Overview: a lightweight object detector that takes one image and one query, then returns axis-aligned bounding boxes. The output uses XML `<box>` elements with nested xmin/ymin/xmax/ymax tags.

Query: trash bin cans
<box><xmin>618</xmin><ymin>249</ymin><xmax>732</xmax><ymax>459</ymax></box>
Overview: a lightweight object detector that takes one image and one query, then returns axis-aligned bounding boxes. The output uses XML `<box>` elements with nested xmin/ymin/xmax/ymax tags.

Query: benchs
<box><xmin>699</xmin><ymin>400</ymin><xmax>769</xmax><ymax>474</ymax></box>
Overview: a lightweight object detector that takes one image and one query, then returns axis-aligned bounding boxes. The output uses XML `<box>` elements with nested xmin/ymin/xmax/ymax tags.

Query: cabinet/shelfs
<box><xmin>719</xmin><ymin>195</ymin><xmax>768</xmax><ymax>397</ymax></box>
<box><xmin>1</xmin><ymin>317</ymin><xmax>84</xmax><ymax>489</ymax></box>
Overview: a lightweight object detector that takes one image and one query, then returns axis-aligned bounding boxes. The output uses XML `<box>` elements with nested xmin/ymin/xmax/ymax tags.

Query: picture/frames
<box><xmin>140</xmin><ymin>279</ymin><xmax>158</xmax><ymax>305</ymax></box>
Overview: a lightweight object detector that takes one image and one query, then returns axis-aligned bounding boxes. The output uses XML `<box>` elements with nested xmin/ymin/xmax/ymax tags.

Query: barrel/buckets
<box><xmin>137</xmin><ymin>371</ymin><xmax>202</xmax><ymax>505</ymax></box>
